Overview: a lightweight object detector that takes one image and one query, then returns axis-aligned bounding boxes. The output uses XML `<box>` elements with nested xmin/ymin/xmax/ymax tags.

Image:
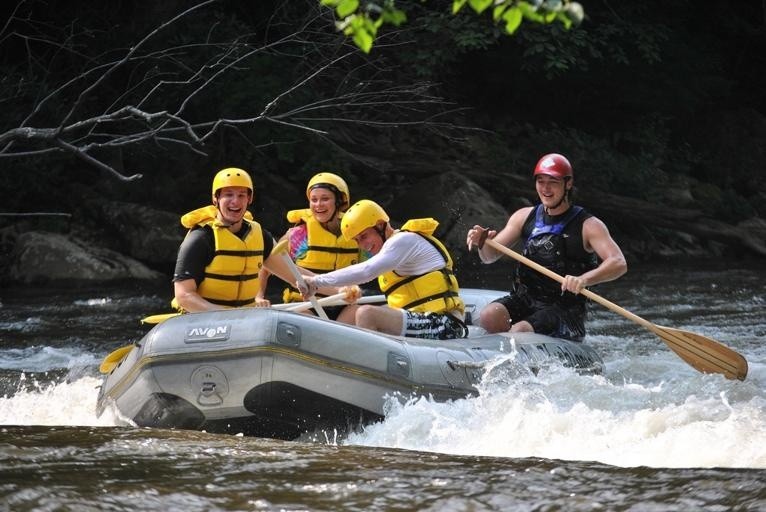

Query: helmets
<box><xmin>212</xmin><ymin>168</ymin><xmax>253</xmax><ymax>199</ymax></box>
<box><xmin>306</xmin><ymin>172</ymin><xmax>390</xmax><ymax>240</ymax></box>
<box><xmin>533</xmin><ymin>153</ymin><xmax>573</xmax><ymax>179</ymax></box>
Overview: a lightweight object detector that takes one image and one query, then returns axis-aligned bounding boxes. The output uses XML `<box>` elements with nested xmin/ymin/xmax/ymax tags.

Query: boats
<box><xmin>94</xmin><ymin>289</ymin><xmax>604</xmax><ymax>437</ymax></box>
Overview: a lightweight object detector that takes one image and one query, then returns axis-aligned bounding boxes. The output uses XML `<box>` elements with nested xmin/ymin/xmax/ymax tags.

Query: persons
<box><xmin>295</xmin><ymin>199</ymin><xmax>468</xmax><ymax>339</ymax></box>
<box><xmin>254</xmin><ymin>172</ymin><xmax>362</xmax><ymax>326</ymax></box>
<box><xmin>172</xmin><ymin>168</ymin><xmax>361</xmax><ymax>314</ymax></box>
<box><xmin>465</xmin><ymin>153</ymin><xmax>628</xmax><ymax>341</ymax></box>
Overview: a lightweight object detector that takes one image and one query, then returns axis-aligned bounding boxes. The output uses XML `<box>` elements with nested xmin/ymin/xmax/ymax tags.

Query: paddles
<box><xmin>100</xmin><ymin>287</ymin><xmax>356</xmax><ymax>373</ymax></box>
<box><xmin>479</xmin><ymin>227</ymin><xmax>747</xmax><ymax>382</ymax></box>
<box><xmin>140</xmin><ymin>288</ymin><xmax>387</xmax><ymax>324</ymax></box>
